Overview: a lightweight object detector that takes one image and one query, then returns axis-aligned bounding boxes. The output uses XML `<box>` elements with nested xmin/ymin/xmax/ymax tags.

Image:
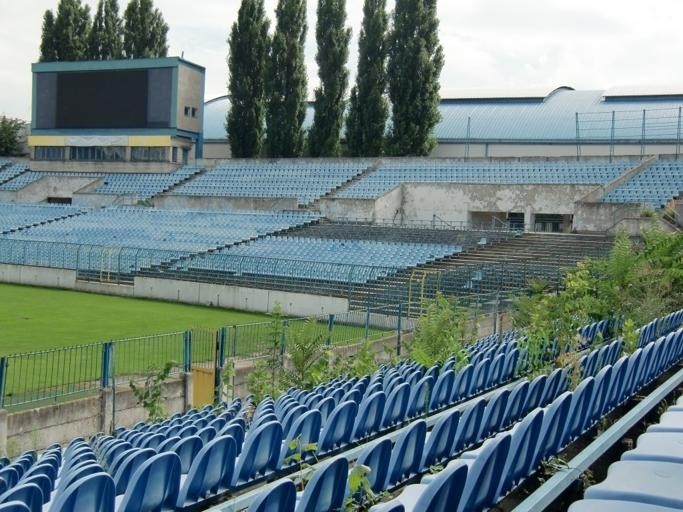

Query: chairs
<box><xmin>1</xmin><ymin>153</ymin><xmax>683</xmax><ymax>323</ymax></box>
<box><xmin>0</xmin><ymin>324</ymin><xmax>682</xmax><ymax>511</ymax></box>
